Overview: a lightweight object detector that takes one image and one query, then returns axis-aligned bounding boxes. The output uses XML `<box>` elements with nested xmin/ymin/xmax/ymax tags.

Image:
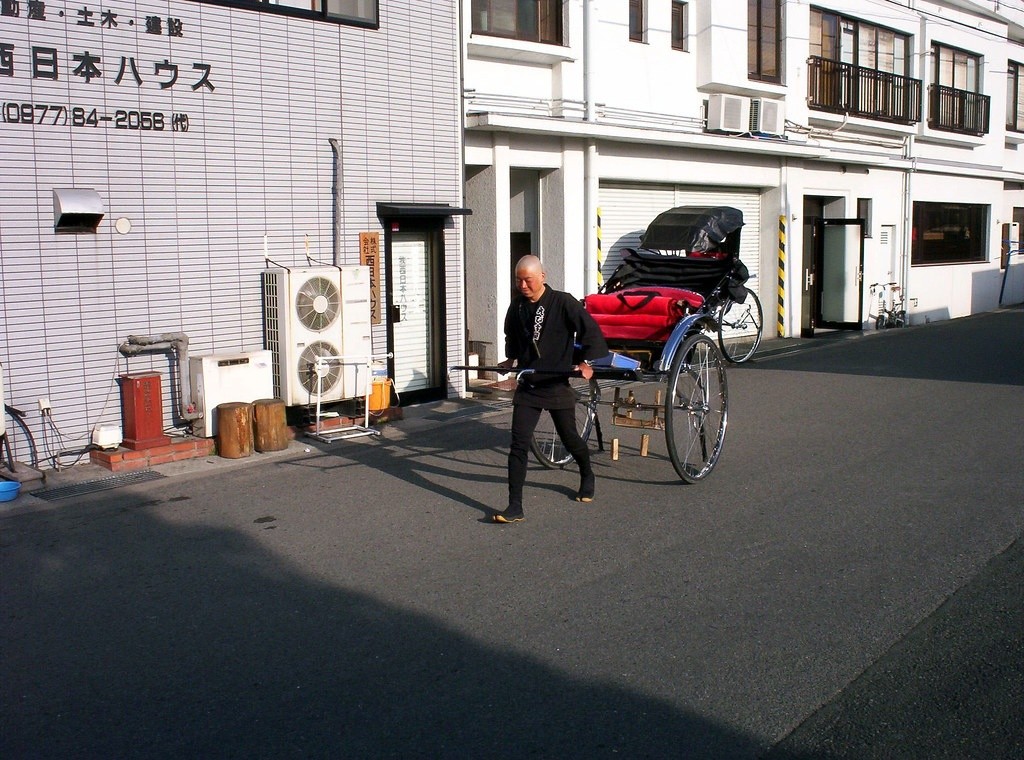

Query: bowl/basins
<box><xmin>0</xmin><ymin>481</ymin><xmax>21</xmax><ymax>502</ymax></box>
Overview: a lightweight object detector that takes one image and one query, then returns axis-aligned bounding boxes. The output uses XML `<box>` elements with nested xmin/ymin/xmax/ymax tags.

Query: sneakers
<box><xmin>492</xmin><ymin>503</ymin><xmax>527</xmax><ymax>523</ymax></box>
<box><xmin>575</xmin><ymin>470</ymin><xmax>595</xmax><ymax>502</ymax></box>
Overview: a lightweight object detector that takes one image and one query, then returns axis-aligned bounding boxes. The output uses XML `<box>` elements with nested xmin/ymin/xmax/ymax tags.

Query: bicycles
<box><xmin>867</xmin><ymin>281</ymin><xmax>907</xmax><ymax>330</ymax></box>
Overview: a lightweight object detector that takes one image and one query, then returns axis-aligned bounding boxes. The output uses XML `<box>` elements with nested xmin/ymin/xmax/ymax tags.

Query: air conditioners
<box><xmin>750</xmin><ymin>98</ymin><xmax>785</xmax><ymax>135</ymax></box>
<box><xmin>708</xmin><ymin>93</ymin><xmax>750</xmax><ymax>133</ymax></box>
<box><xmin>262</xmin><ymin>267</ymin><xmax>372</xmax><ymax>407</ymax></box>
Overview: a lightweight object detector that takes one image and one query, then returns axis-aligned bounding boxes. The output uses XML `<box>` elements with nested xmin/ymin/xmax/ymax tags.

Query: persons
<box><xmin>491</xmin><ymin>255</ymin><xmax>609</xmax><ymax>522</ymax></box>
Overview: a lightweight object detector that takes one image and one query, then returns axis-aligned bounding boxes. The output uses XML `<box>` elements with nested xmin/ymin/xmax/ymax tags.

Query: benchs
<box><xmin>572</xmin><ymin>247</ymin><xmax>734</xmax><ymax>362</ymax></box>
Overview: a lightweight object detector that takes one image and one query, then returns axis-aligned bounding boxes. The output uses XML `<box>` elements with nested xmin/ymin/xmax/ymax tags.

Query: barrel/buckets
<box><xmin>362</xmin><ymin>380</ymin><xmax>391</xmax><ymax>410</ymax></box>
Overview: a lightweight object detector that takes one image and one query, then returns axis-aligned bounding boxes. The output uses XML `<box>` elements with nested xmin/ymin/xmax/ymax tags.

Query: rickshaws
<box><xmin>449</xmin><ymin>203</ymin><xmax>761</xmax><ymax>488</ymax></box>
